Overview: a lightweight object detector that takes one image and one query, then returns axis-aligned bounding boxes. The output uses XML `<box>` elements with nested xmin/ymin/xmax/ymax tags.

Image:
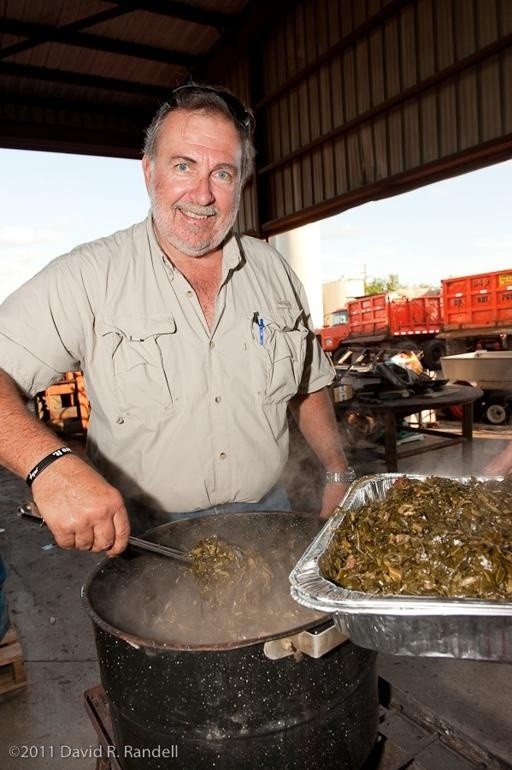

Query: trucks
<box><xmin>34</xmin><ymin>379</ymin><xmax>86</xmax><ymax>444</ymax></box>
<box><xmin>315</xmin><ymin>269</ymin><xmax>512</xmax><ymax>370</ymax></box>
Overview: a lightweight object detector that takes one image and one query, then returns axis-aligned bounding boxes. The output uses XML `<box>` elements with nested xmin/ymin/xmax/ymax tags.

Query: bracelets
<box><xmin>27</xmin><ymin>446</ymin><xmax>74</xmax><ymax>489</ymax></box>
<box><xmin>320</xmin><ymin>467</ymin><xmax>355</xmax><ymax>484</ymax></box>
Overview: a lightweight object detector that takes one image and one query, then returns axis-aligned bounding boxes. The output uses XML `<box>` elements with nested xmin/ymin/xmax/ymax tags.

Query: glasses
<box><xmin>163</xmin><ymin>86</ymin><xmax>255</xmax><ymax>131</ymax></box>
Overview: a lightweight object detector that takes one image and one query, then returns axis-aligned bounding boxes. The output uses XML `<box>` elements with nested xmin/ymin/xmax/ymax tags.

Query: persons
<box><xmin>1</xmin><ymin>83</ymin><xmax>356</xmax><ymax>560</ymax></box>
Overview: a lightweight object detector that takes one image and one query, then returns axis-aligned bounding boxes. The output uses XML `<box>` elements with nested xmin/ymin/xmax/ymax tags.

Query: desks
<box><xmin>336</xmin><ymin>383</ymin><xmax>485</xmax><ymax>475</ymax></box>
